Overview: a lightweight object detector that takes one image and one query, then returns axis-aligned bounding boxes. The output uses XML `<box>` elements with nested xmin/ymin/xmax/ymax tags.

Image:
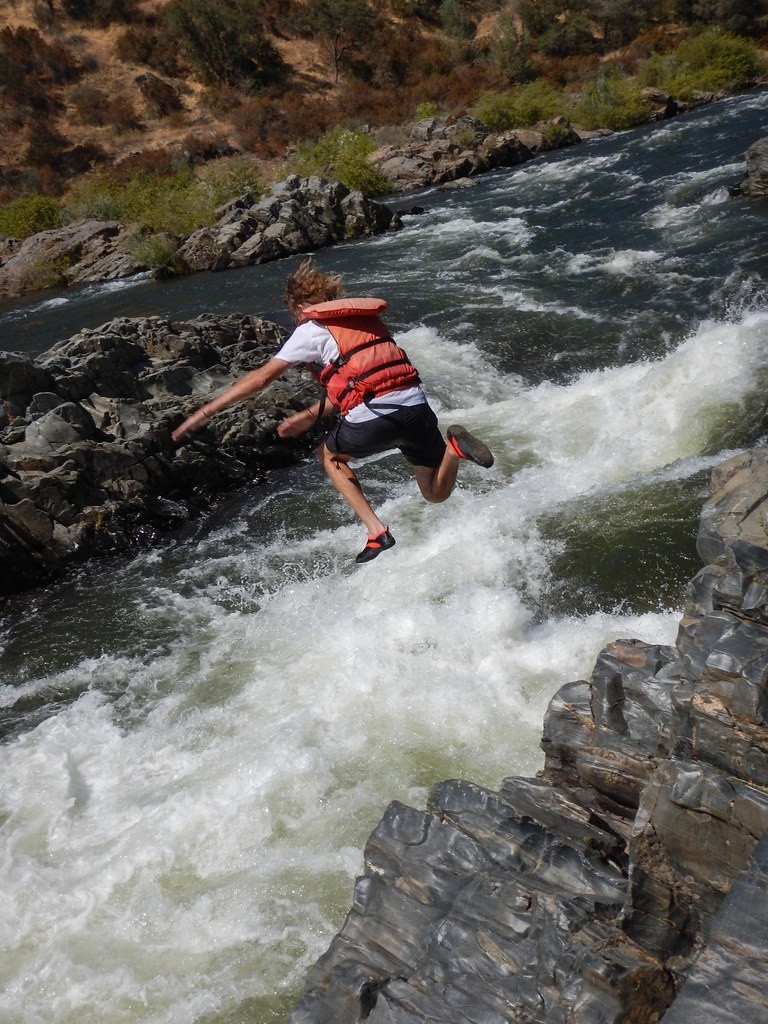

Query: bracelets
<box><xmin>308</xmin><ymin>408</ymin><xmax>315</xmax><ymax>417</ymax></box>
<box><xmin>201</xmin><ymin>409</ymin><xmax>209</xmax><ymax>419</ymax></box>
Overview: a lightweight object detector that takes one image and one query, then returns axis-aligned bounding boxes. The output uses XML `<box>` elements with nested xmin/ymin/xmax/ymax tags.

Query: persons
<box><xmin>171</xmin><ymin>258</ymin><xmax>494</xmax><ymax>563</ymax></box>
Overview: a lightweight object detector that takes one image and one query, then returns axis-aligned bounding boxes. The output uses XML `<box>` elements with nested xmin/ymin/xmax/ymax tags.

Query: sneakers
<box><xmin>355</xmin><ymin>527</ymin><xmax>396</xmax><ymax>563</ymax></box>
<box><xmin>447</xmin><ymin>425</ymin><xmax>494</xmax><ymax>469</ymax></box>
